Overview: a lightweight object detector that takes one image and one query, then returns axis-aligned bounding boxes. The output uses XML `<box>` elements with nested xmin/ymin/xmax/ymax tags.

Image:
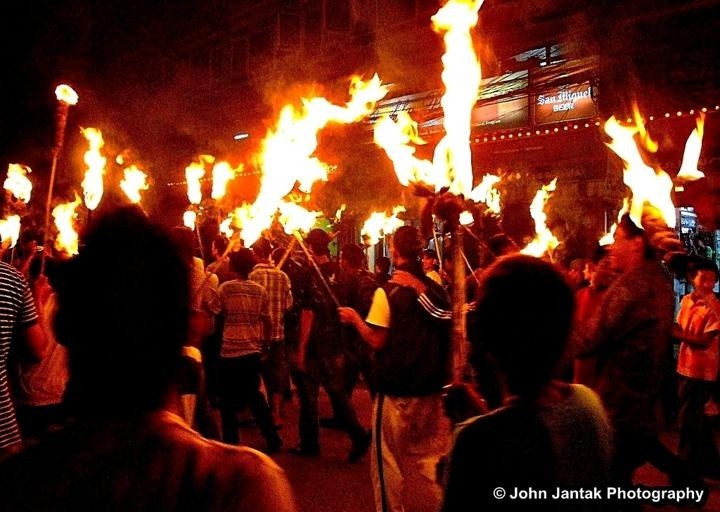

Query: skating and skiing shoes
<box><xmin>319</xmin><ymin>416</ymin><xmax>338</xmax><ymax>428</ymax></box>
<box><xmin>285</xmin><ymin>446</ymin><xmax>320</xmax><ymax>456</ymax></box>
<box><xmin>349</xmin><ymin>429</ymin><xmax>372</xmax><ymax>462</ymax></box>
